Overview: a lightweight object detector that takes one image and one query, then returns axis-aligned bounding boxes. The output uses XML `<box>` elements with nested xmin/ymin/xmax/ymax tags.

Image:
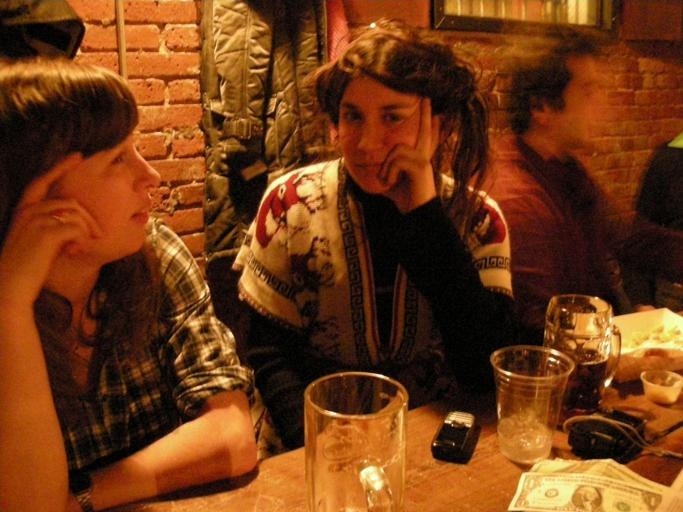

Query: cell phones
<box><xmin>431</xmin><ymin>410</ymin><xmax>476</xmax><ymax>461</ymax></box>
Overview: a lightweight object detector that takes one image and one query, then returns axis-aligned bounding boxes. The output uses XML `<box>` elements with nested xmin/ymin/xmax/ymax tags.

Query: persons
<box><xmin>0</xmin><ymin>58</ymin><xmax>263</xmax><ymax>509</ymax></box>
<box><xmin>226</xmin><ymin>16</ymin><xmax>518</xmax><ymax>459</ymax></box>
<box><xmin>486</xmin><ymin>20</ymin><xmax>683</xmax><ymax>345</ymax></box>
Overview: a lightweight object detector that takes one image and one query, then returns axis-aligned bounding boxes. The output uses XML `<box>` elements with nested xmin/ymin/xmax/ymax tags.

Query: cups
<box><xmin>542</xmin><ymin>294</ymin><xmax>623</xmax><ymax>425</ymax></box>
<box><xmin>489</xmin><ymin>343</ymin><xmax>576</xmax><ymax>465</ymax></box>
<box><xmin>301</xmin><ymin>370</ymin><xmax>410</xmax><ymax>512</ymax></box>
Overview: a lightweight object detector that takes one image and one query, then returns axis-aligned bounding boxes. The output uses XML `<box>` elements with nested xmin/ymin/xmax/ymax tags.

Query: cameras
<box><xmin>567</xmin><ymin>409</ymin><xmax>645</xmax><ymax>462</ymax></box>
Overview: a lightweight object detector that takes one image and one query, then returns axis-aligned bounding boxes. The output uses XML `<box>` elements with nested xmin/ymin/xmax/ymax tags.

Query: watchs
<box><xmin>63</xmin><ymin>468</ymin><xmax>96</xmax><ymax>511</ymax></box>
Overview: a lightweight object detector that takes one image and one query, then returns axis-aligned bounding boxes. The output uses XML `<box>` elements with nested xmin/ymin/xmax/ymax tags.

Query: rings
<box><xmin>44</xmin><ymin>207</ymin><xmax>74</xmax><ymax>229</ymax></box>
